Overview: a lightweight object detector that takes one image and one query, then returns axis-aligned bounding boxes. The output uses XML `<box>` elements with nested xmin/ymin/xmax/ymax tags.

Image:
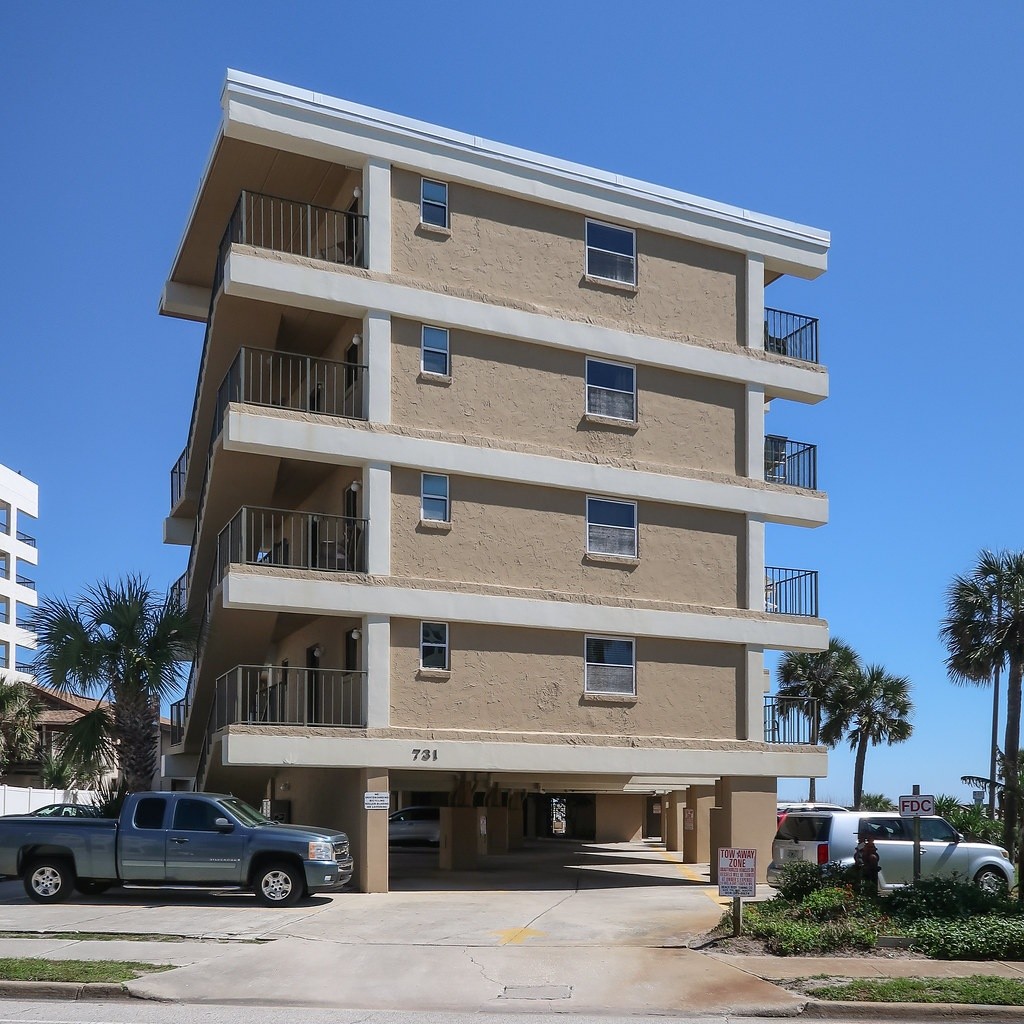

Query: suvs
<box><xmin>766</xmin><ymin>811</ymin><xmax>1015</xmax><ymax>906</ymax></box>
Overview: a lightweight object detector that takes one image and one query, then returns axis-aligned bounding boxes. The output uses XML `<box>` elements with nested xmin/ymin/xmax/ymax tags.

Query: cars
<box><xmin>388</xmin><ymin>807</ymin><xmax>441</xmax><ymax>841</ymax></box>
<box><xmin>776</xmin><ymin>800</ymin><xmax>849</xmax><ymax>820</ymax></box>
<box><xmin>1</xmin><ymin>803</ymin><xmax>101</xmax><ymax>818</ymax></box>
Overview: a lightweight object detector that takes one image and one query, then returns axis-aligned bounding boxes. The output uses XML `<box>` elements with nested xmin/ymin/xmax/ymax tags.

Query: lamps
<box><xmin>351</xmin><ymin>479</ymin><xmax>363</xmax><ymax>491</ymax></box>
<box><xmin>280</xmin><ymin>781</ymin><xmax>291</xmax><ymax>792</ymax></box>
<box><xmin>314</xmin><ymin>513</ymin><xmax>328</xmax><ymax>521</ymax></box>
<box><xmin>351</xmin><ymin>627</ymin><xmax>362</xmax><ymax>639</ymax></box>
<box><xmin>352</xmin><ymin>333</ymin><xmax>363</xmax><ymax>345</ymax></box>
<box><xmin>353</xmin><ymin>185</ymin><xmax>362</xmax><ymax>197</ymax></box>
<box><xmin>314</xmin><ymin>646</ymin><xmax>326</xmax><ymax>657</ymax></box>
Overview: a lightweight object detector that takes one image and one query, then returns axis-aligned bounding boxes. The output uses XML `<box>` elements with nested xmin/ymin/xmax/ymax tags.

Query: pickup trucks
<box><xmin>1</xmin><ymin>792</ymin><xmax>354</xmax><ymax>908</ymax></box>
